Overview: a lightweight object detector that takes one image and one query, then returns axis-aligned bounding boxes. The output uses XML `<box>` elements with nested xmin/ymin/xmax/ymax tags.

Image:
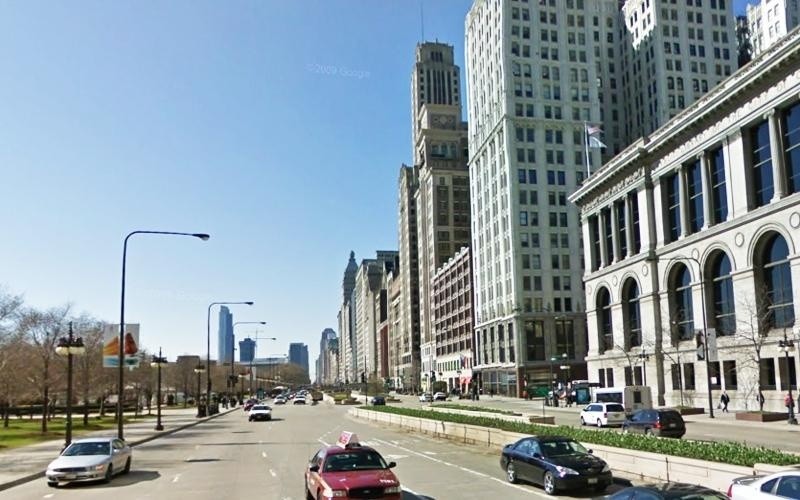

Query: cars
<box><xmin>586</xmin><ymin>480</ymin><xmax>735</xmax><ymax>500</ymax></box>
<box><xmin>45</xmin><ymin>436</ymin><xmax>131</xmax><ymax>487</ymax></box>
<box><xmin>434</xmin><ymin>392</ymin><xmax>447</xmax><ymax>402</ymax></box>
<box><xmin>243</xmin><ymin>384</ymin><xmax>308</xmax><ymax>412</ymax></box>
<box><xmin>418</xmin><ymin>393</ymin><xmax>435</xmax><ymax>402</ymax></box>
<box><xmin>371</xmin><ymin>396</ymin><xmax>386</xmax><ymax>405</ymax></box>
<box><xmin>246</xmin><ymin>403</ymin><xmax>272</xmax><ymax>422</ymax></box>
<box><xmin>303</xmin><ymin>431</ymin><xmax>404</xmax><ymax>500</ymax></box>
<box><xmin>497</xmin><ymin>433</ymin><xmax>615</xmax><ymax>496</ymax></box>
<box><xmin>548</xmin><ymin>385</ymin><xmax>566</xmax><ymax>398</ymax></box>
<box><xmin>726</xmin><ymin>471</ymin><xmax>800</xmax><ymax>500</ymax></box>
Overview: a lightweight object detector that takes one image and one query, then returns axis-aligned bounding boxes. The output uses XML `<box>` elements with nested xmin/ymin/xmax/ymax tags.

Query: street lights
<box><xmin>637</xmin><ymin>350</ymin><xmax>650</xmax><ymax>387</ymax></box>
<box><xmin>56</xmin><ymin>318</ymin><xmax>86</xmax><ymax>455</ymax></box>
<box><xmin>645</xmin><ymin>255</ymin><xmax>717</xmax><ymax>419</ymax></box>
<box><xmin>227</xmin><ymin>318</ymin><xmax>288</xmax><ymax>407</ymax></box>
<box><xmin>149</xmin><ymin>345</ymin><xmax>166</xmax><ymax>432</ymax></box>
<box><xmin>409</xmin><ymin>371</ymin><xmax>463</xmax><ymax>398</ymax></box>
<box><xmin>114</xmin><ymin>230</ymin><xmax>212</xmax><ymax>440</ymax></box>
<box><xmin>511</xmin><ymin>306</ymin><xmax>563</xmax><ymax>409</ymax></box>
<box><xmin>776</xmin><ymin>333</ymin><xmax>799</xmax><ymax>425</ymax></box>
<box><xmin>551</xmin><ymin>352</ymin><xmax>567</xmax><ymax>392</ymax></box>
<box><xmin>205</xmin><ymin>299</ymin><xmax>254</xmax><ymax>416</ymax></box>
<box><xmin>660</xmin><ymin>320</ymin><xmax>685</xmax><ymax>407</ymax></box>
<box><xmin>194</xmin><ymin>358</ymin><xmax>207</xmax><ymax>419</ymax></box>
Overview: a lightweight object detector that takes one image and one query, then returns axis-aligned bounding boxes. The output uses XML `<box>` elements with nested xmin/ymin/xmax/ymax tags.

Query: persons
<box><xmin>565</xmin><ymin>391</ymin><xmax>572</xmax><ymax>407</ymax></box>
<box><xmin>757</xmin><ymin>391</ymin><xmax>765</xmax><ymax>411</ymax></box>
<box><xmin>784</xmin><ymin>391</ymin><xmax>795</xmax><ymax>416</ymax></box>
<box><xmin>720</xmin><ymin>390</ymin><xmax>730</xmax><ymax>412</ymax></box>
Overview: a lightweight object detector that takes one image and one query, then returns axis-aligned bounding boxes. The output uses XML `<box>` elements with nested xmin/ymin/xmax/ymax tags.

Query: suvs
<box><xmin>619</xmin><ymin>406</ymin><xmax>691</xmax><ymax>438</ymax></box>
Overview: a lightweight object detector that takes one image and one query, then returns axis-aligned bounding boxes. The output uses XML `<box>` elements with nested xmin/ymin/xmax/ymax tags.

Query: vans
<box><xmin>578</xmin><ymin>401</ymin><xmax>626</xmax><ymax>429</ymax></box>
